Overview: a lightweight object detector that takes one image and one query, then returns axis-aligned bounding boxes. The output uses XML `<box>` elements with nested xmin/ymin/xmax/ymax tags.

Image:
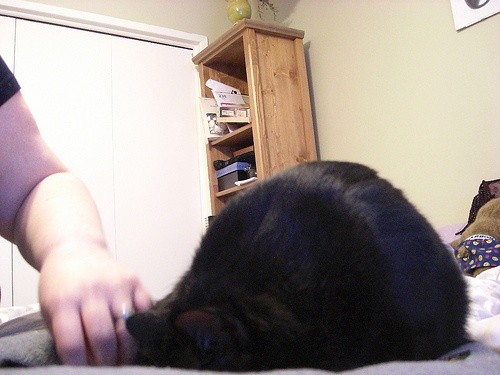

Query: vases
<box><xmin>225</xmin><ymin>0</ymin><xmax>251</xmax><ymax>23</ymax></box>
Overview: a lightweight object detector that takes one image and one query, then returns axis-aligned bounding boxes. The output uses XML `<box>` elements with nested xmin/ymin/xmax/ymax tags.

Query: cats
<box><xmin>122</xmin><ymin>155</ymin><xmax>477</xmax><ymax>374</ymax></box>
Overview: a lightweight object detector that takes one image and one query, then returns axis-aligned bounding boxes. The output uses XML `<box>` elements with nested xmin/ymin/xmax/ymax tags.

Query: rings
<box><xmin>114</xmin><ymin>304</ymin><xmax>134</xmax><ymax>321</ymax></box>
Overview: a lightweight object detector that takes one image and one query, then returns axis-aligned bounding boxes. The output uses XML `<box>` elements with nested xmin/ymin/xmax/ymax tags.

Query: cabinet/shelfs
<box><xmin>0</xmin><ymin>0</ymin><xmax>208</xmax><ymax>308</ymax></box>
<box><xmin>192</xmin><ymin>17</ymin><xmax>318</xmax><ymax>217</ymax></box>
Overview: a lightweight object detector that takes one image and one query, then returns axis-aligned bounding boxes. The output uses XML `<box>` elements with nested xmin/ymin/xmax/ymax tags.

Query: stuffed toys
<box><xmin>447</xmin><ymin>198</ymin><xmax>500</xmax><ymax>278</ymax></box>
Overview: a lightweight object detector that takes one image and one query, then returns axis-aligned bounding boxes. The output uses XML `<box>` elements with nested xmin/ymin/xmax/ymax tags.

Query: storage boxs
<box><xmin>216</xmin><ymin>162</ymin><xmax>256</xmax><ymax>192</ymax></box>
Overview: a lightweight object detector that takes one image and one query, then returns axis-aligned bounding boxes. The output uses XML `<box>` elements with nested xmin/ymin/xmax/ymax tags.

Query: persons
<box><xmin>0</xmin><ymin>56</ymin><xmax>151</xmax><ymax>367</ymax></box>
